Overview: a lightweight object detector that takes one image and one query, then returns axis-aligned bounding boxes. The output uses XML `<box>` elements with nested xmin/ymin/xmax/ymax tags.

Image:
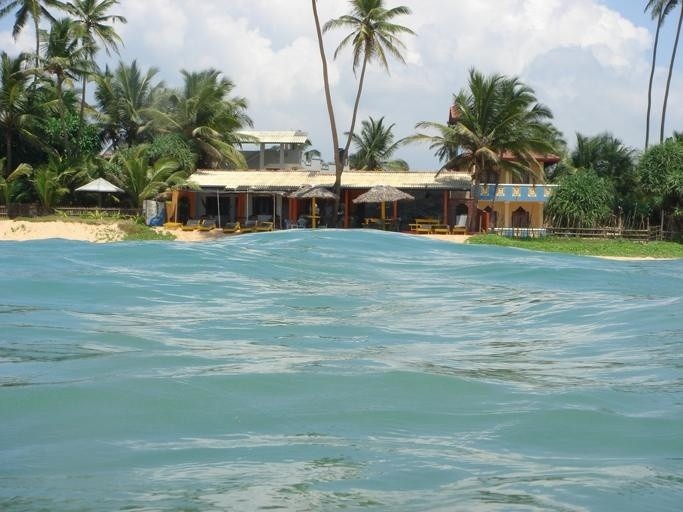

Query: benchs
<box><xmin>408</xmin><ymin>224</ymin><xmax>450</xmax><ymax>231</ymax></box>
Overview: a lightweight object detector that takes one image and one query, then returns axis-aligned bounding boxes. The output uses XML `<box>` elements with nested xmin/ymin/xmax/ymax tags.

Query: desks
<box><xmin>415</xmin><ymin>218</ymin><xmax>440</xmax><ymax>229</ymax></box>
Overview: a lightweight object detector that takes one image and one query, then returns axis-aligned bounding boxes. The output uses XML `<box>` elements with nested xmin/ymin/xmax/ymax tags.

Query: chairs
<box><xmin>181</xmin><ymin>219</ymin><xmax>273</xmax><ymax>232</ymax></box>
<box><xmin>416</xmin><ymin>224</ymin><xmax>468</xmax><ymax>235</ymax></box>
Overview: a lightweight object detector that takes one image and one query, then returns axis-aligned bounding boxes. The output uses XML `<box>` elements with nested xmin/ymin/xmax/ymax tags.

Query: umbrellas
<box><xmin>285</xmin><ymin>184</ymin><xmax>340</xmax><ymax>228</ymax></box>
<box><xmin>75</xmin><ymin>176</ymin><xmax>126</xmax><ymax>207</ymax></box>
<box><xmin>352</xmin><ymin>183</ymin><xmax>414</xmax><ymax>221</ymax></box>
